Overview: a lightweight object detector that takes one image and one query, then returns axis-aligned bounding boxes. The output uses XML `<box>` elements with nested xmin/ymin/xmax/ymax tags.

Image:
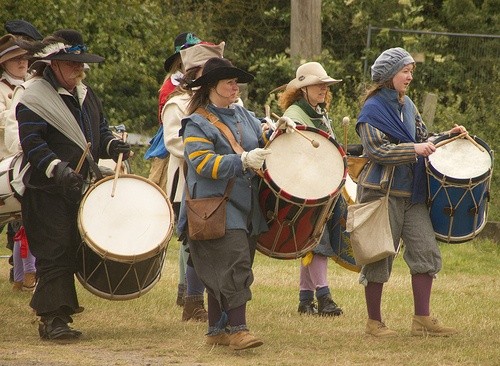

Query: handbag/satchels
<box><xmin>185</xmin><ymin>197</ymin><xmax>224</xmax><ymax>241</ymax></box>
<box><xmin>345</xmin><ymin>197</ymin><xmax>395</xmax><ymax>264</ymax></box>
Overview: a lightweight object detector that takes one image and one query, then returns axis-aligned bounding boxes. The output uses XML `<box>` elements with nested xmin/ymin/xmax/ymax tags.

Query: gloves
<box><xmin>111</xmin><ymin>139</ymin><xmax>130</xmax><ymax>161</ymax></box>
<box><xmin>274</xmin><ymin>116</ymin><xmax>296</xmax><ymax>134</ymax></box>
<box><xmin>54</xmin><ymin>163</ymin><xmax>84</xmax><ymax>204</ymax></box>
<box><xmin>239</xmin><ymin>147</ymin><xmax>270</xmax><ymax>170</ymax></box>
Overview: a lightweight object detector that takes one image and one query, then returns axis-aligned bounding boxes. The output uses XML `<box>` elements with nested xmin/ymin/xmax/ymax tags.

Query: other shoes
<box><xmin>38</xmin><ymin>320</ymin><xmax>82</xmax><ymax>341</ymax></box>
<box><xmin>12</xmin><ymin>281</ymin><xmax>22</xmax><ymax>292</ymax></box>
<box><xmin>229</xmin><ymin>329</ymin><xmax>263</xmax><ymax>349</ymax></box>
<box><xmin>22</xmin><ymin>273</ymin><xmax>36</xmax><ymax>292</ymax></box>
<box><xmin>206</xmin><ymin>332</ymin><xmax>231</xmax><ymax>347</ymax></box>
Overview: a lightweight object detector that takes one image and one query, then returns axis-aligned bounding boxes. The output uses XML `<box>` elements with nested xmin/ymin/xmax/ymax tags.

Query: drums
<box><xmin>251</xmin><ymin>125</ymin><xmax>347</xmax><ymax>261</ymax></box>
<box><xmin>75</xmin><ymin>173</ymin><xmax>175</xmax><ymax>302</ymax></box>
<box><xmin>423</xmin><ymin>132</ymin><xmax>496</xmax><ymax>243</ymax></box>
<box><xmin>0</xmin><ymin>150</ymin><xmax>25</xmax><ymax>226</ymax></box>
<box><xmin>326</xmin><ymin>155</ymin><xmax>366</xmax><ymax>273</ymax></box>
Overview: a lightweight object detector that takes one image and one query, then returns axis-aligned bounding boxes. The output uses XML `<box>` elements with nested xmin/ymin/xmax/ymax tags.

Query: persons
<box><xmin>178</xmin><ymin>57</ymin><xmax>297</xmax><ymax>350</ymax></box>
<box><xmin>356</xmin><ymin>47</ymin><xmax>470</xmax><ymax>337</ymax></box>
<box><xmin>0</xmin><ymin>34</ymin><xmax>39</xmax><ymax>284</ymax></box>
<box><xmin>15</xmin><ymin>30</ymin><xmax>131</xmax><ymax>345</ymax></box>
<box><xmin>4</xmin><ymin>20</ymin><xmax>46</xmax><ymax>293</ymax></box>
<box><xmin>160</xmin><ymin>32</ymin><xmax>216</xmax><ymax>307</ymax></box>
<box><xmin>276</xmin><ymin>60</ymin><xmax>343</xmax><ymax>316</ymax></box>
<box><xmin>158</xmin><ymin>40</ymin><xmax>226</xmax><ymax>323</ymax></box>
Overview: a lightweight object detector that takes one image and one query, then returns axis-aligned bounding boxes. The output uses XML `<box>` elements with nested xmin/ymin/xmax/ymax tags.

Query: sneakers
<box><xmin>410</xmin><ymin>315</ymin><xmax>455</xmax><ymax>337</ymax></box>
<box><xmin>365</xmin><ymin>318</ymin><xmax>397</xmax><ymax>337</ymax></box>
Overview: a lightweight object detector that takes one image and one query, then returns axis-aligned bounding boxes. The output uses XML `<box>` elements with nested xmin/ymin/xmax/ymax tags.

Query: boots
<box><xmin>297</xmin><ymin>298</ymin><xmax>318</xmax><ymax>314</ymax></box>
<box><xmin>317</xmin><ymin>294</ymin><xmax>342</xmax><ymax>315</ymax></box>
<box><xmin>182</xmin><ymin>295</ymin><xmax>208</xmax><ymax>323</ymax></box>
<box><xmin>177</xmin><ymin>284</ymin><xmax>187</xmax><ymax>307</ymax></box>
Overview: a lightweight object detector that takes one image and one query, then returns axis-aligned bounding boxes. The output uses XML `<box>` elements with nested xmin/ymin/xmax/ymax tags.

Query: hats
<box><xmin>0</xmin><ymin>33</ymin><xmax>27</xmax><ymax>65</ymax></box>
<box><xmin>371</xmin><ymin>47</ymin><xmax>415</xmax><ymax>84</ymax></box>
<box><xmin>32</xmin><ymin>29</ymin><xmax>105</xmax><ymax>63</ymax></box>
<box><xmin>185</xmin><ymin>57</ymin><xmax>254</xmax><ymax>88</ymax></box>
<box><xmin>164</xmin><ymin>33</ymin><xmax>203</xmax><ymax>71</ymax></box>
<box><xmin>6</xmin><ymin>21</ymin><xmax>42</xmax><ymax>40</ymax></box>
<box><xmin>179</xmin><ymin>41</ymin><xmax>225</xmax><ymax>72</ymax></box>
<box><xmin>286</xmin><ymin>61</ymin><xmax>343</xmax><ymax>93</ymax></box>
